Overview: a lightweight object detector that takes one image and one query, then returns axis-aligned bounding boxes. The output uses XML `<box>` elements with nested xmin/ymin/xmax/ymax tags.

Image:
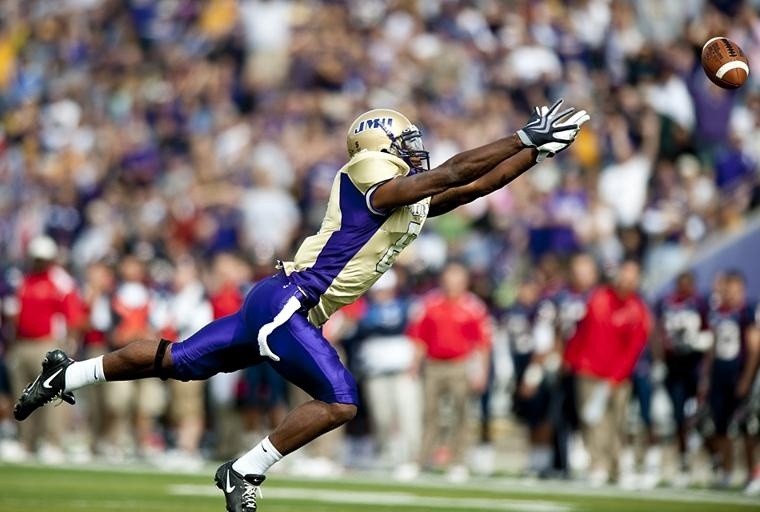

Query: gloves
<box><xmin>515</xmin><ymin>98</ymin><xmax>590</xmax><ymax>165</ymax></box>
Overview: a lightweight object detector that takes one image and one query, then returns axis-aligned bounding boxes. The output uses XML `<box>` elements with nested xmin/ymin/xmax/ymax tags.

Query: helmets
<box><xmin>346</xmin><ymin>108</ymin><xmax>432</xmax><ymax>174</ymax></box>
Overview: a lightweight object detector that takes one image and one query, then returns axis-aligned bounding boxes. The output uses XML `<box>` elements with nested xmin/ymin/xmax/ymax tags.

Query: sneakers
<box><xmin>13</xmin><ymin>349</ymin><xmax>76</xmax><ymax>422</ymax></box>
<box><xmin>213</xmin><ymin>458</ymin><xmax>266</xmax><ymax>512</ymax></box>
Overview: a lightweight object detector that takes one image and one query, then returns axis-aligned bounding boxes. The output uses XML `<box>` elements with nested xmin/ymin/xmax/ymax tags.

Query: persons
<box><xmin>1</xmin><ymin>1</ymin><xmax>760</xmax><ymax>495</ymax></box>
<box><xmin>9</xmin><ymin>95</ymin><xmax>591</xmax><ymax>512</ymax></box>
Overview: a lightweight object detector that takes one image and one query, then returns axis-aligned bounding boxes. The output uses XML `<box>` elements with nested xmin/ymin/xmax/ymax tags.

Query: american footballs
<box><xmin>700</xmin><ymin>36</ymin><xmax>748</xmax><ymax>89</ymax></box>
